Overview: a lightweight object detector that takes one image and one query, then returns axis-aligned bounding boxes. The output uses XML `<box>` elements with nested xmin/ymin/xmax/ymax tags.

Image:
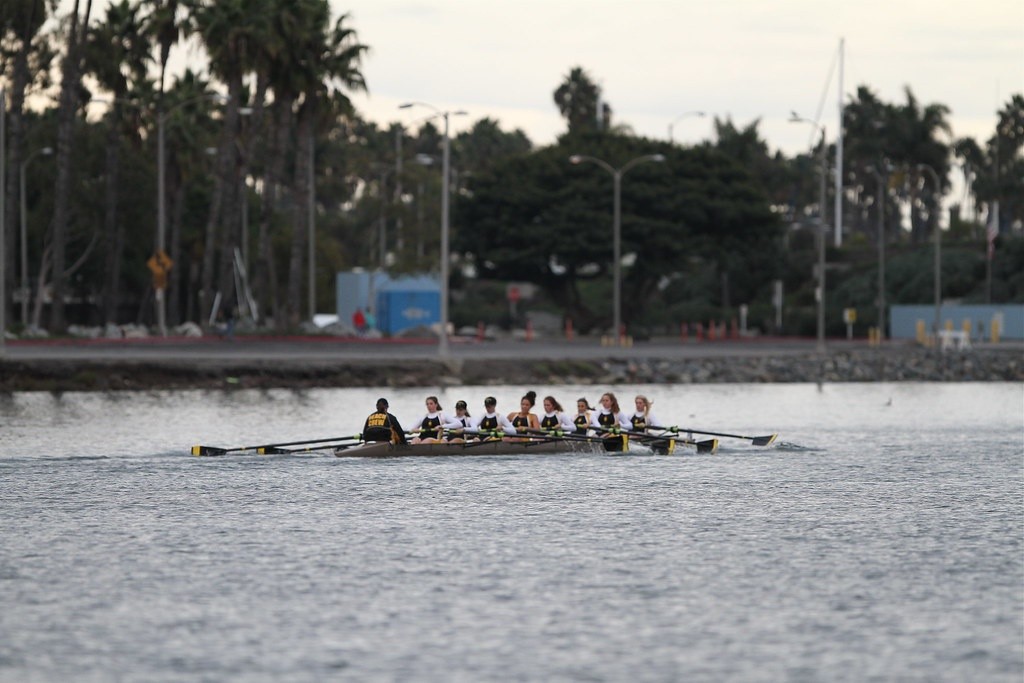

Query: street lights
<box><xmin>785</xmin><ymin>112</ymin><xmax>832</xmax><ymax>354</ymax></box>
<box><xmin>917</xmin><ymin>161</ymin><xmax>941</xmax><ymax>337</ymax></box>
<box><xmin>18</xmin><ymin>144</ymin><xmax>57</xmax><ymax>332</ymax></box>
<box><xmin>565</xmin><ymin>151</ymin><xmax>664</xmax><ymax>345</ymax></box>
<box><xmin>394</xmin><ymin>100</ymin><xmax>452</xmax><ymax>350</ymax></box>
<box><xmin>152</xmin><ymin>93</ymin><xmax>233</xmax><ymax>338</ymax></box>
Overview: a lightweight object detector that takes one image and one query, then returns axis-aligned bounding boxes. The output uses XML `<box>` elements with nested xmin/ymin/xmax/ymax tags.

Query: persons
<box><xmin>588</xmin><ymin>392</ymin><xmax>634</xmax><ymax>438</ymax></box>
<box><xmin>624</xmin><ymin>396</ymin><xmax>663</xmax><ymax>440</ymax></box>
<box><xmin>408</xmin><ymin>397</ymin><xmax>463</xmax><ymax>444</ymax></box>
<box><xmin>362</xmin><ymin>307</ymin><xmax>378</xmax><ymax>329</ymax></box>
<box><xmin>360</xmin><ymin>398</ymin><xmax>408</xmax><ymax>444</ymax></box>
<box><xmin>352</xmin><ymin>306</ymin><xmax>366</xmax><ymax>338</ymax></box>
<box><xmin>568</xmin><ymin>397</ymin><xmax>602</xmax><ymax>438</ymax></box>
<box><xmin>539</xmin><ymin>395</ymin><xmax>577</xmax><ymax>436</ymax></box>
<box><xmin>473</xmin><ymin>397</ymin><xmax>517</xmax><ymax>443</ymax></box>
<box><xmin>438</xmin><ymin>400</ymin><xmax>477</xmax><ymax>444</ymax></box>
<box><xmin>506</xmin><ymin>390</ymin><xmax>541</xmax><ymax>437</ymax></box>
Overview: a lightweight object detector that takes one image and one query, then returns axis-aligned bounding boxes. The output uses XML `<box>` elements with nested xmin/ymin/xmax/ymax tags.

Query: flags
<box><xmin>986</xmin><ymin>200</ymin><xmax>1000</xmax><ymax>243</ymax></box>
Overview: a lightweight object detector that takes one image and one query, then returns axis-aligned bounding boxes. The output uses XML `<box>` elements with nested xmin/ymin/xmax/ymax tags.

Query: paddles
<box><xmin>599</xmin><ymin>424</ymin><xmax>620</xmax><ymax>428</ymax></box>
<box><xmin>517</xmin><ymin>427</ymin><xmax>676</xmax><ymax>456</ymax></box>
<box><xmin>404</xmin><ymin>427</ymin><xmax>504</xmax><ymax>446</ymax></box>
<box><xmin>579</xmin><ymin>422</ymin><xmax>718</xmax><ymax>454</ymax></box>
<box><xmin>445</xmin><ymin>428</ymin><xmax>629</xmax><ymax>458</ymax></box>
<box><xmin>189</xmin><ymin>434</ymin><xmax>374</xmax><ymax>457</ymax></box>
<box><xmin>541</xmin><ymin>425</ymin><xmax>558</xmax><ymax>430</ymax></box>
<box><xmin>255</xmin><ymin>428</ymin><xmax>439</xmax><ymax>454</ymax></box>
<box><xmin>632</xmin><ymin>422</ymin><xmax>778</xmax><ymax>446</ymax></box>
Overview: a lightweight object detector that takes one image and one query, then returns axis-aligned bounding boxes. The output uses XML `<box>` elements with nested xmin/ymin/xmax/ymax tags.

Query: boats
<box><xmin>332</xmin><ymin>428</ymin><xmax>668</xmax><ymax>459</ymax></box>
